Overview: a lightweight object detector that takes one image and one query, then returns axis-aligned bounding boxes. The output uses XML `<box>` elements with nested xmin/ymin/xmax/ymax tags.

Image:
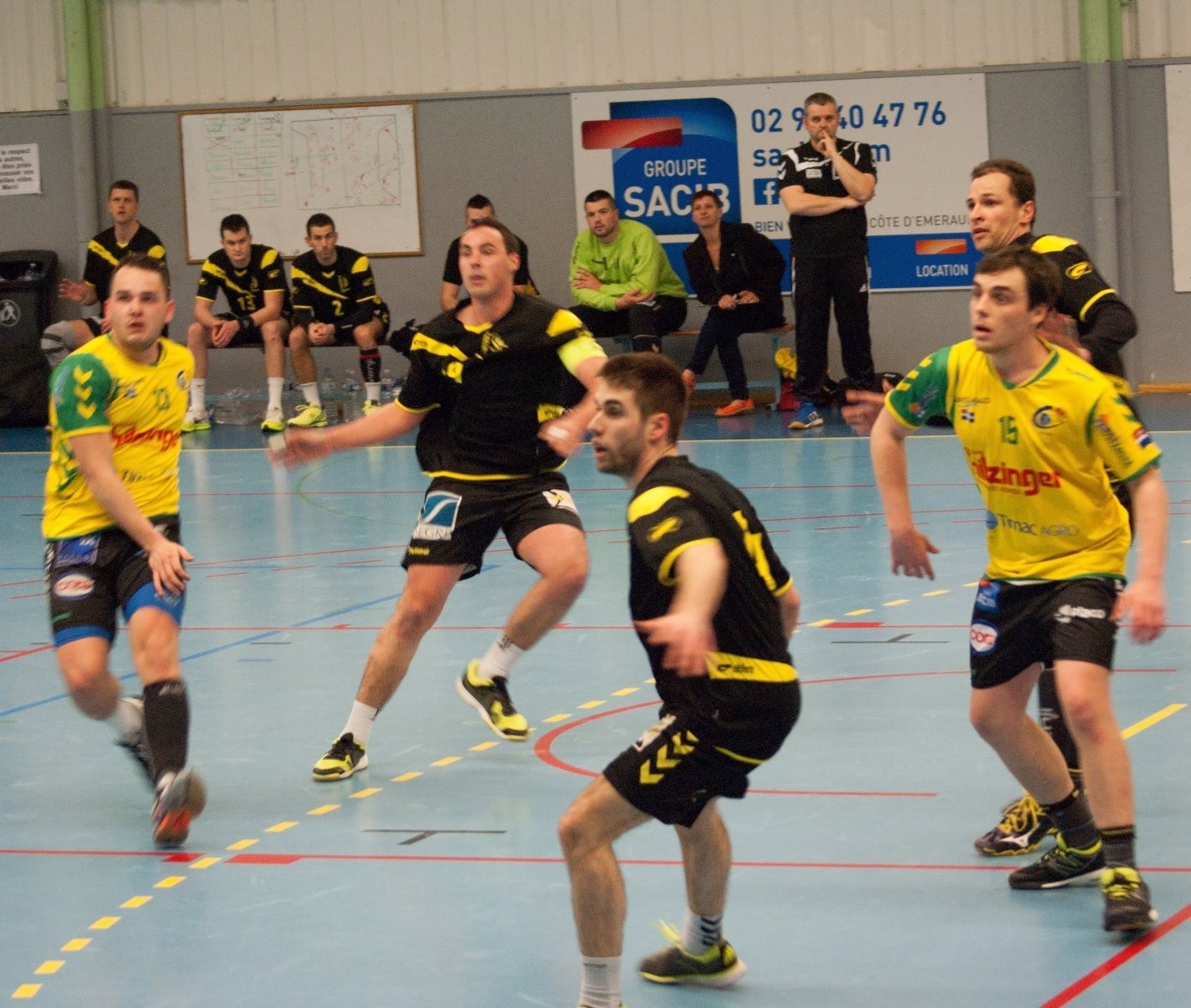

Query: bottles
<box><xmin>282</xmin><ymin>378</ymin><xmax>307</xmax><ymax>419</ymax></box>
<box><xmin>319</xmin><ymin>367</ymin><xmax>339</xmax><ymax>425</ymax></box>
<box><xmin>380</xmin><ymin>368</ymin><xmax>404</xmax><ymax>406</ymax></box>
<box><xmin>25</xmin><ymin>263</ymin><xmax>40</xmax><ymax>281</ymax></box>
<box><xmin>341</xmin><ymin>369</ymin><xmax>364</xmax><ymax>422</ymax></box>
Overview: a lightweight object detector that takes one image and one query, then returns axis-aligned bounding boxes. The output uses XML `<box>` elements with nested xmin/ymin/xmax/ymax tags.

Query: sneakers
<box><xmin>1009</xmin><ymin>831</ymin><xmax>1102</xmax><ymax>890</ymax></box>
<box><xmin>682</xmin><ymin>375</ymin><xmax>695</xmax><ymax>395</ymax></box>
<box><xmin>312</xmin><ymin>732</ymin><xmax>369</xmax><ymax>781</ymax></box>
<box><xmin>716</xmin><ymin>399</ymin><xmax>753</xmax><ymax>415</ymax></box>
<box><xmin>362</xmin><ymin>399</ymin><xmax>381</xmax><ymax>415</ymax></box>
<box><xmin>261</xmin><ymin>407</ymin><xmax>285</xmax><ymax>430</ymax></box>
<box><xmin>113</xmin><ymin>696</ymin><xmax>157</xmax><ymax>784</ymax></box>
<box><xmin>638</xmin><ymin>919</ymin><xmax>743</xmax><ymax>985</ymax></box>
<box><xmin>455</xmin><ymin>659</ymin><xmax>530</xmax><ymax>742</ymax></box>
<box><xmin>1099</xmin><ymin>864</ymin><xmax>1149</xmax><ymax>931</ymax></box>
<box><xmin>788</xmin><ymin>403</ymin><xmax>823</xmax><ymax>428</ymax></box>
<box><xmin>287</xmin><ymin>402</ymin><xmax>329</xmax><ymax>427</ymax></box>
<box><xmin>975</xmin><ymin>790</ymin><xmax>1059</xmax><ymax>857</ymax></box>
<box><xmin>180</xmin><ymin>411</ymin><xmax>210</xmax><ymax>432</ymax></box>
<box><xmin>151</xmin><ymin>770</ymin><xmax>204</xmax><ymax>849</ymax></box>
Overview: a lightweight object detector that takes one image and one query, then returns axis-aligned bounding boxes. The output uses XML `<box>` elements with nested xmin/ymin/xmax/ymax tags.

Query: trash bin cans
<box><xmin>0</xmin><ymin>250</ymin><xmax>60</xmax><ymax>428</ymax></box>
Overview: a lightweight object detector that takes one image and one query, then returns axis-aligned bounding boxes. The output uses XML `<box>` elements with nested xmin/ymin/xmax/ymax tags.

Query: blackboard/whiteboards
<box><xmin>178</xmin><ymin>101</ymin><xmax>424</xmax><ymax>263</ymax></box>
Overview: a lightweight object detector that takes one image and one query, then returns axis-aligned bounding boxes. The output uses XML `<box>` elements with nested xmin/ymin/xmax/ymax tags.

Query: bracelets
<box><xmin>237</xmin><ymin>315</ymin><xmax>254</xmax><ymax>332</ymax></box>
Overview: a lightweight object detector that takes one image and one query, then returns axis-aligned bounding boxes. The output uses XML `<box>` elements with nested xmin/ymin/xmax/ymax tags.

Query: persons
<box><xmin>560</xmin><ymin>352</ymin><xmax>803</xmax><ymax>1007</ymax></box>
<box><xmin>286</xmin><ymin>213</ymin><xmax>391</xmax><ymax>427</ymax></box>
<box><xmin>833</xmin><ymin>160</ymin><xmax>1142</xmax><ymax>855</ymax></box>
<box><xmin>180</xmin><ymin>213</ymin><xmax>293</xmax><ymax>434</ymax></box>
<box><xmin>682</xmin><ymin>190</ymin><xmax>787</xmax><ymax>415</ymax></box>
<box><xmin>776</xmin><ymin>92</ymin><xmax>879</xmax><ymax>429</ymax></box>
<box><xmin>268</xmin><ymin>218</ymin><xmax>607</xmax><ymax>781</ymax></box>
<box><xmin>42</xmin><ymin>252</ymin><xmax>209</xmax><ymax>854</ymax></box>
<box><xmin>566</xmin><ymin>189</ymin><xmax>687</xmax><ymax>352</ymax></box>
<box><xmin>442</xmin><ymin>195</ymin><xmax>541</xmax><ymax>311</ymax></box>
<box><xmin>869</xmin><ymin>242</ymin><xmax>1169</xmax><ymax>929</ymax></box>
<box><xmin>42</xmin><ymin>178</ymin><xmax>171</xmax><ymax>376</ymax></box>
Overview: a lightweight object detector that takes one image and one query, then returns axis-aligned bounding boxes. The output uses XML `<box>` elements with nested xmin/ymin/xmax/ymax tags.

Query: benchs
<box><xmin>208</xmin><ymin>331</ymin><xmax>396</xmax><ymax>356</ymax></box>
<box><xmin>613</xmin><ymin>321</ymin><xmax>796</xmax><ymax>414</ymax></box>
